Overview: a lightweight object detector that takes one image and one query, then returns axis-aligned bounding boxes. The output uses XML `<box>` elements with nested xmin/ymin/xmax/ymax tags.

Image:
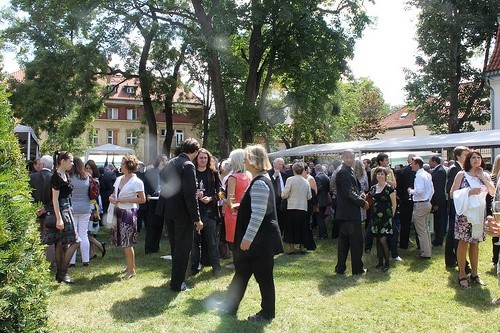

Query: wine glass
<box><xmin>408</xmin><ymin>187</ymin><xmax>412</xmax><ymax>200</ymax></box>
<box><xmin>491</xmin><ymin>200</ymin><xmax>500</xmax><ymax>246</ymax></box>
<box><xmin>229</xmin><ymin>194</ymin><xmax>237</xmax><ymax>215</ymax></box>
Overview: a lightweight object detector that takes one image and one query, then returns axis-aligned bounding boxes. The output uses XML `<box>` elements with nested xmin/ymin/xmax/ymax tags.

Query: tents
<box><xmin>85</xmin><ymin>143</ymin><xmax>136</xmax><ymax>164</ymax></box>
<box><xmin>267</xmin><ymin>129</ymin><xmax>500</xmax><ymax>157</ymax></box>
<box><xmin>14</xmin><ymin>123</ymin><xmax>40</xmax><ymax>160</ymax></box>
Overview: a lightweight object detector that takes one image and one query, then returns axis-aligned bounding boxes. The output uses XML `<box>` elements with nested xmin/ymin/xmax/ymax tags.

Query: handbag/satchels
<box><xmin>89</xmin><ymin>176</ymin><xmax>99</xmax><ymax>200</ymax></box>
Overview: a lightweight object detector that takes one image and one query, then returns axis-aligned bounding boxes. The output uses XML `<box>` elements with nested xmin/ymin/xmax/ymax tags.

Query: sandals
<box><xmin>470</xmin><ymin>274</ymin><xmax>486</xmax><ymax>286</ymax></box>
<box><xmin>458</xmin><ymin>276</ymin><xmax>469</xmax><ymax>289</ymax></box>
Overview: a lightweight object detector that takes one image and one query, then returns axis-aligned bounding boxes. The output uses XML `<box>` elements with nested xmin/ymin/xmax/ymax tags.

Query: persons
<box><xmin>26</xmin><ymin>150</ymin><xmax>81</xmax><ymax>286</ymax></box>
<box><xmin>268</xmin><ymin>157</ymin><xmax>341</xmax><ymax>256</ymax></box>
<box><xmin>391</xmin><ymin>152</ymin><xmax>456</xmax><ymax>262</ymax></box>
<box><xmin>109</xmin><ymin>156</ymin><xmax>145</xmax><ymax>280</ymax></box>
<box><xmin>214</xmin><ymin>143</ymin><xmax>284</xmax><ymax>321</ymax></box>
<box><xmin>66</xmin><ymin>156</ymin><xmax>106</xmax><ymax>268</ymax></box>
<box><xmin>445</xmin><ymin>145</ymin><xmax>496</xmax><ymax>288</ymax></box>
<box><xmin>136</xmin><ymin>152</ymin><xmax>169</xmax><ymax>255</ymax></box>
<box><xmin>97</xmin><ymin>162</ymin><xmax>120</xmax><ymax>227</ymax></box>
<box><xmin>159</xmin><ymin>138</ymin><xmax>231</xmax><ymax>292</ymax></box>
<box><xmin>330</xmin><ymin>148</ymin><xmax>396</xmax><ymax>275</ymax></box>
<box><xmin>484</xmin><ymin>153</ymin><xmax>500</xmax><ymax>306</ymax></box>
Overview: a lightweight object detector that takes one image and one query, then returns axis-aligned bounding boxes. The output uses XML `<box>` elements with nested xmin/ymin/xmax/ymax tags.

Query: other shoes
<box><xmin>287</xmin><ymin>251</ymin><xmax>295</xmax><ymax>254</ymax></box>
<box><xmin>248</xmin><ymin>314</ymin><xmax>265</xmax><ymax>320</ymax></box>
<box><xmin>190</xmin><ymin>269</ymin><xmax>199</xmax><ymax>275</ymax></box>
<box><xmin>182</xmin><ymin>286</ymin><xmax>192</xmax><ymax>291</ymax></box>
<box><xmin>366</xmin><ymin>249</ymin><xmax>371</xmax><ymax>254</ymax></box>
<box><xmin>298</xmin><ymin>250</ymin><xmax>304</xmax><ymax>254</ymax></box>
<box><xmin>376</xmin><ymin>264</ymin><xmax>388</xmax><ymax>271</ymax></box>
<box><xmin>121</xmin><ymin>268</ymin><xmax>136</xmax><ymax>280</ymax></box>
<box><xmin>69</xmin><ymin>263</ymin><xmax>75</xmax><ymax>267</ymax></box>
<box><xmin>432</xmin><ymin>240</ymin><xmax>442</xmax><ymax>246</ymax></box>
<box><xmin>394</xmin><ymin>256</ymin><xmax>403</xmax><ymax>261</ymax></box>
<box><xmin>213</xmin><ymin>270</ymin><xmax>222</xmax><ymax>275</ymax></box>
<box><xmin>465</xmin><ymin>266</ymin><xmax>472</xmax><ymax>274</ymax></box>
<box><xmin>84</xmin><ymin>262</ymin><xmax>90</xmax><ymax>266</ymax></box>
<box><xmin>213</xmin><ymin>300</ymin><xmax>238</xmax><ymax>314</ymax></box>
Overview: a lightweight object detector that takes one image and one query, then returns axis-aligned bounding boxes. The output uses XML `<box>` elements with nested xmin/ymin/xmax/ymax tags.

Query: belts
<box><xmin>413</xmin><ymin>199</ymin><xmax>429</xmax><ymax>203</ymax></box>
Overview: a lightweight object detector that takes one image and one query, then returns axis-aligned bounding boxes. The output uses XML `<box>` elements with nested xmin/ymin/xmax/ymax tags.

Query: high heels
<box><xmin>89</xmin><ymin>254</ymin><xmax>97</xmax><ymax>260</ymax></box>
<box><xmin>56</xmin><ymin>273</ymin><xmax>74</xmax><ymax>285</ymax></box>
<box><xmin>101</xmin><ymin>242</ymin><xmax>108</xmax><ymax>257</ymax></box>
